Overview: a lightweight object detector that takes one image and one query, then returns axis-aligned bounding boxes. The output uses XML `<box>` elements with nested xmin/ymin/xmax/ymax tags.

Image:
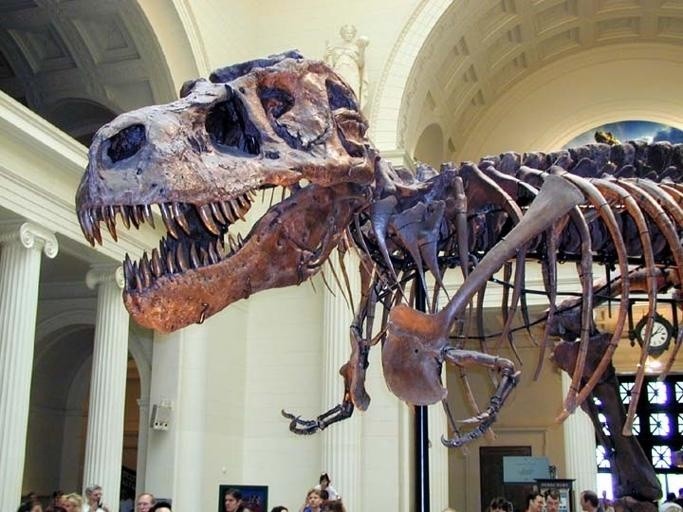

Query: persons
<box><xmin>662</xmin><ymin>488</ymin><xmax>683</xmax><ymax>508</ymax></box>
<box><xmin>324</xmin><ymin>25</ymin><xmax>369</xmax><ymax>103</ymax></box>
<box><xmin>225</xmin><ymin>472</ymin><xmax>341</xmax><ymax>512</ymax></box>
<box><xmin>17</xmin><ymin>484</ymin><xmax>171</xmax><ymax>512</ymax></box>
<box><xmin>489</xmin><ymin>487</ymin><xmax>616</xmax><ymax>512</ymax></box>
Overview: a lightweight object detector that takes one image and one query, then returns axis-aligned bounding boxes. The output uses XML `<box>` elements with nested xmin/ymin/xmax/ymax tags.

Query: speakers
<box><xmin>148</xmin><ymin>404</ymin><xmax>172</xmax><ymax>432</ymax></box>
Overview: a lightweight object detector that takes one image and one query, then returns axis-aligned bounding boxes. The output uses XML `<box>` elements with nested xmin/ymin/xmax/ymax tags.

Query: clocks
<box><xmin>635</xmin><ymin>312</ymin><xmax>673</xmax><ymax>358</ymax></box>
